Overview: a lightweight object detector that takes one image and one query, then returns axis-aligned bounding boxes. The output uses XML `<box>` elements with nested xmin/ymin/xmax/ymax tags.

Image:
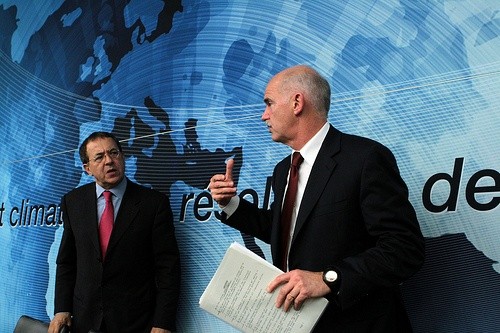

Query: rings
<box><xmin>289</xmin><ymin>293</ymin><xmax>295</xmax><ymax>299</ymax></box>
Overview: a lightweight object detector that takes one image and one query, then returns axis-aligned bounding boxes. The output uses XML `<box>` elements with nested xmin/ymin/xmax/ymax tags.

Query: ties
<box><xmin>278</xmin><ymin>151</ymin><xmax>305</xmax><ymax>272</ymax></box>
<box><xmin>97</xmin><ymin>191</ymin><xmax>115</xmax><ymax>260</ymax></box>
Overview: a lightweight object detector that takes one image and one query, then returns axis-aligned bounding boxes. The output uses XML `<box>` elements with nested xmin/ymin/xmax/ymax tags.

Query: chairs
<box><xmin>12</xmin><ymin>315</ymin><xmax>71</xmax><ymax>333</ymax></box>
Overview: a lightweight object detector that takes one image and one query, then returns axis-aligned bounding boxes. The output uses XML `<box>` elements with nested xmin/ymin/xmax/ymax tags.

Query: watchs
<box><xmin>322</xmin><ymin>265</ymin><xmax>342</xmax><ymax>292</ymax></box>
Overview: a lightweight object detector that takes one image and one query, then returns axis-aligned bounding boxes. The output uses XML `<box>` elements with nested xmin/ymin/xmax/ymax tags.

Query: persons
<box><xmin>207</xmin><ymin>63</ymin><xmax>427</xmax><ymax>332</ymax></box>
<box><xmin>47</xmin><ymin>130</ymin><xmax>182</xmax><ymax>333</ymax></box>
